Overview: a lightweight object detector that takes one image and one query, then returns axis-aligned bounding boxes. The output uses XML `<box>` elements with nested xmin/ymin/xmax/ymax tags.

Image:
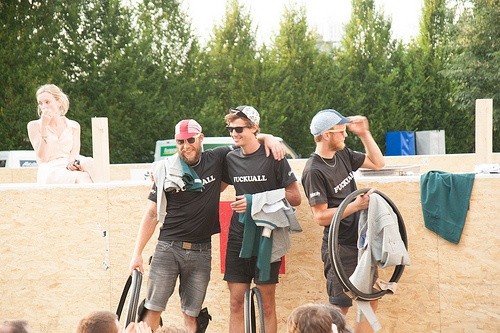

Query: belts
<box><xmin>166</xmin><ymin>240</ymin><xmax>211</xmax><ymax>251</ymax></box>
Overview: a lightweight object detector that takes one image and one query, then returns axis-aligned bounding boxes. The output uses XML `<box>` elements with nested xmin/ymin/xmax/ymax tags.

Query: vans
<box><xmin>0</xmin><ymin>151</ymin><xmax>39</xmax><ymax>167</ymax></box>
<box><xmin>153</xmin><ymin>134</ymin><xmax>299</xmax><ymax>161</ymax></box>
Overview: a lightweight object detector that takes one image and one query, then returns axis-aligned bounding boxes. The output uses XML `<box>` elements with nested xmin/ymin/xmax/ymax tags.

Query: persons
<box><xmin>77</xmin><ymin>311</ymin><xmax>152</xmax><ymax>333</ymax></box>
<box><xmin>0</xmin><ymin>320</ymin><xmax>28</xmax><ymax>333</ymax></box>
<box><xmin>143</xmin><ymin>105</ymin><xmax>302</xmax><ymax>333</ymax></box>
<box><xmin>287</xmin><ymin>304</ymin><xmax>346</xmax><ymax>333</ymax></box>
<box><xmin>129</xmin><ymin>119</ymin><xmax>286</xmax><ymax>333</ymax></box>
<box><xmin>27</xmin><ymin>84</ymin><xmax>99</xmax><ymax>183</ymax></box>
<box><xmin>301</xmin><ymin>109</ymin><xmax>385</xmax><ymax>333</ymax></box>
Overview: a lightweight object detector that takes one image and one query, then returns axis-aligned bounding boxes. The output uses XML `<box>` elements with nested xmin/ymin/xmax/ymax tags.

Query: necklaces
<box><xmin>192</xmin><ymin>156</ymin><xmax>201</xmax><ymax>167</ymax></box>
<box><xmin>316</xmin><ymin>152</ymin><xmax>336</xmax><ymax>167</ymax></box>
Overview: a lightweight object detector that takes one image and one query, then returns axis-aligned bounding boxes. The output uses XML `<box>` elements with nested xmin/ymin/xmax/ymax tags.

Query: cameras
<box><xmin>66</xmin><ymin>160</ymin><xmax>80</xmax><ymax>170</ymax></box>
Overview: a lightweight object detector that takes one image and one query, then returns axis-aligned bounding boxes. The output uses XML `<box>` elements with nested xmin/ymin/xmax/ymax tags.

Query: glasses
<box><xmin>323</xmin><ymin>127</ymin><xmax>346</xmax><ymax>136</ymax></box>
<box><xmin>225</xmin><ymin>124</ymin><xmax>251</xmax><ymax>133</ymax></box>
<box><xmin>175</xmin><ymin>134</ymin><xmax>200</xmax><ymax>145</ymax></box>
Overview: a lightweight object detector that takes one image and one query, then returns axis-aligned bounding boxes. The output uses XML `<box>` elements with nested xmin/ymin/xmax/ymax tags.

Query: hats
<box><xmin>175</xmin><ymin>119</ymin><xmax>202</xmax><ymax>140</ymax></box>
<box><xmin>229</xmin><ymin>105</ymin><xmax>261</xmax><ymax>128</ymax></box>
<box><xmin>310</xmin><ymin>110</ymin><xmax>352</xmax><ymax>137</ymax></box>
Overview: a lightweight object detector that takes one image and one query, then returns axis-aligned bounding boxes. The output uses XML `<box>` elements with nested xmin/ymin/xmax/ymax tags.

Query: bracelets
<box><xmin>40</xmin><ymin>135</ymin><xmax>47</xmax><ymax>144</ymax></box>
